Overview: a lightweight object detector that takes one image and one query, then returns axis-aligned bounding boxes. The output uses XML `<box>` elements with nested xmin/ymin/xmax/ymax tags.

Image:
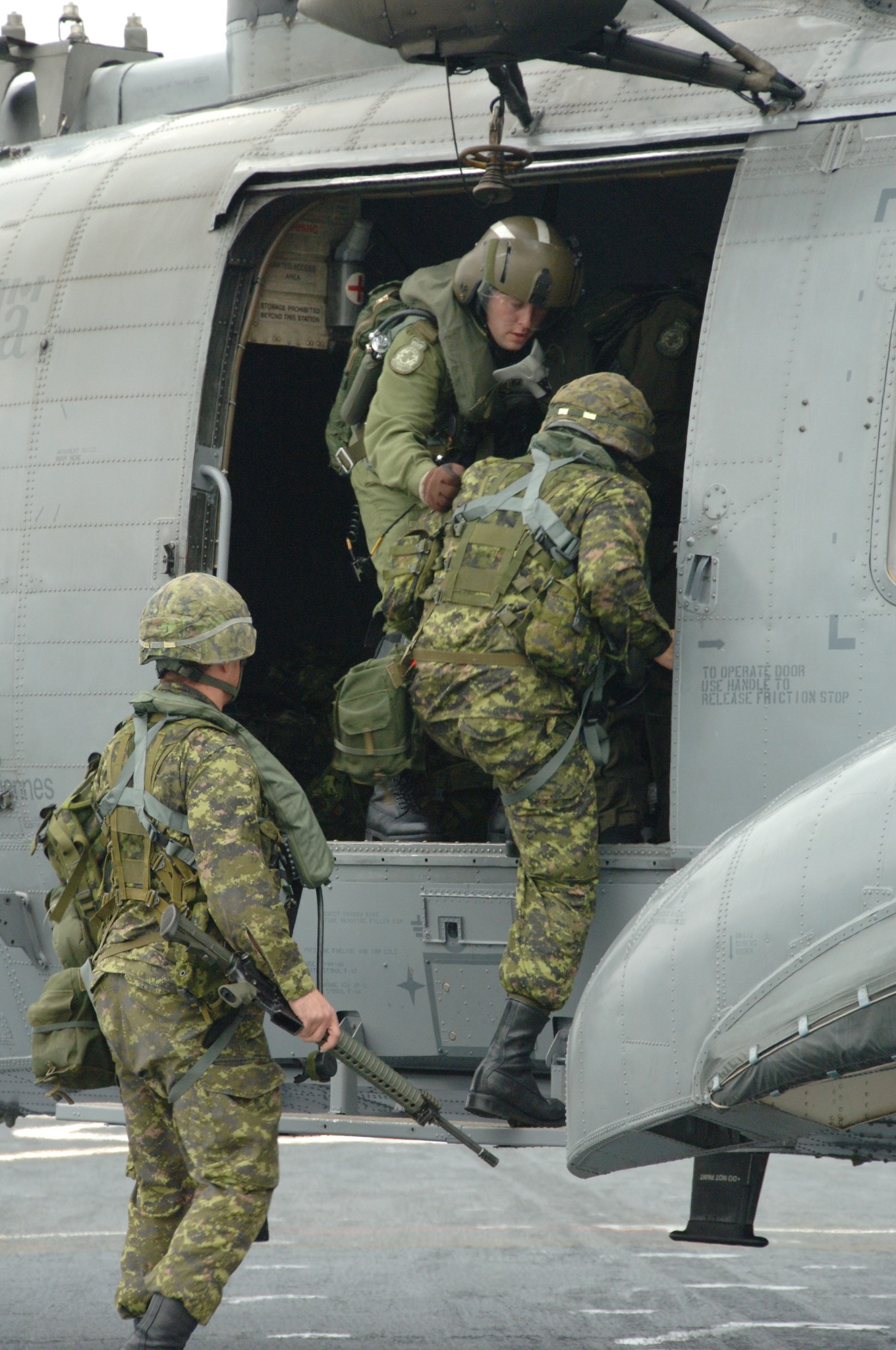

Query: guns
<box><xmin>159</xmin><ymin>902</ymin><xmax>501</xmax><ymax>1169</ymax></box>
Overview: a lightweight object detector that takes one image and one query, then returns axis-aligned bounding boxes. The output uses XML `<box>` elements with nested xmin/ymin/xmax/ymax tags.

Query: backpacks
<box><xmin>535</xmin><ymin>284</ymin><xmax>707</xmax><ymax>415</ymax></box>
<box><xmin>30</xmin><ymin>716</ymin><xmax>136</xmax><ymax>953</ymax></box>
<box><xmin>324</xmin><ymin>280</ymin><xmax>412</xmax><ymax>476</ymax></box>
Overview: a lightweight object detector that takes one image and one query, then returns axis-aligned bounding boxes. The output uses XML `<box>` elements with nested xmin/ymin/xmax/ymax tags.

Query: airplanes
<box><xmin>1</xmin><ymin>1</ymin><xmax>896</xmax><ymax>1258</ymax></box>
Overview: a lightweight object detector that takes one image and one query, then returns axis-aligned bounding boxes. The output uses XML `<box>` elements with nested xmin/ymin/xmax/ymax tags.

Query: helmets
<box><xmin>138</xmin><ymin>572</ymin><xmax>256</xmax><ymax>665</ymax></box>
<box><xmin>539</xmin><ymin>372</ymin><xmax>659</xmax><ymax>469</ymax></box>
<box><xmin>453</xmin><ymin>216</ymin><xmax>584</xmax><ymax>331</ymax></box>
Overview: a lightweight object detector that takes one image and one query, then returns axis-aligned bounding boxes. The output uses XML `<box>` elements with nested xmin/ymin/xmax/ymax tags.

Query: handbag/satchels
<box><xmin>44</xmin><ymin>884</ymin><xmax>97</xmax><ymax>969</ymax></box>
<box><xmin>27</xmin><ymin>967</ymin><xmax>116</xmax><ymax>1104</ymax></box>
<box><xmin>333</xmin><ymin>651</ymin><xmax>427</xmax><ymax>784</ymax></box>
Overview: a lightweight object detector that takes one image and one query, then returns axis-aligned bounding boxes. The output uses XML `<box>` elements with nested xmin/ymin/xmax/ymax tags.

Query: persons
<box><xmin>322</xmin><ymin>215</ymin><xmax>582</xmax><ymax>845</ymax></box>
<box><xmin>80</xmin><ymin>573</ymin><xmax>338</xmax><ymax>1350</ymax></box>
<box><xmin>406</xmin><ymin>370</ymin><xmax>678</xmax><ymax>1132</ymax></box>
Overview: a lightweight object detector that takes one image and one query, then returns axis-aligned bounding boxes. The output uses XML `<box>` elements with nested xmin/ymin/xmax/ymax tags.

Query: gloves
<box><xmin>418</xmin><ymin>463</ymin><xmax>465</xmax><ymax>514</ymax></box>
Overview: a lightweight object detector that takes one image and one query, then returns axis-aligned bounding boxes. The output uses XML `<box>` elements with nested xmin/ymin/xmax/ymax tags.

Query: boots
<box><xmin>465</xmin><ymin>998</ymin><xmax>565</xmax><ymax>1127</ymax></box>
<box><xmin>133</xmin><ymin>1314</ymin><xmax>142</xmax><ymax>1329</ymax></box>
<box><xmin>121</xmin><ymin>1293</ymin><xmax>198</xmax><ymax>1350</ymax></box>
<box><xmin>485</xmin><ymin>797</ymin><xmax>514</xmax><ymax>843</ymax></box>
<box><xmin>364</xmin><ymin>776</ymin><xmax>436</xmax><ymax>842</ymax></box>
